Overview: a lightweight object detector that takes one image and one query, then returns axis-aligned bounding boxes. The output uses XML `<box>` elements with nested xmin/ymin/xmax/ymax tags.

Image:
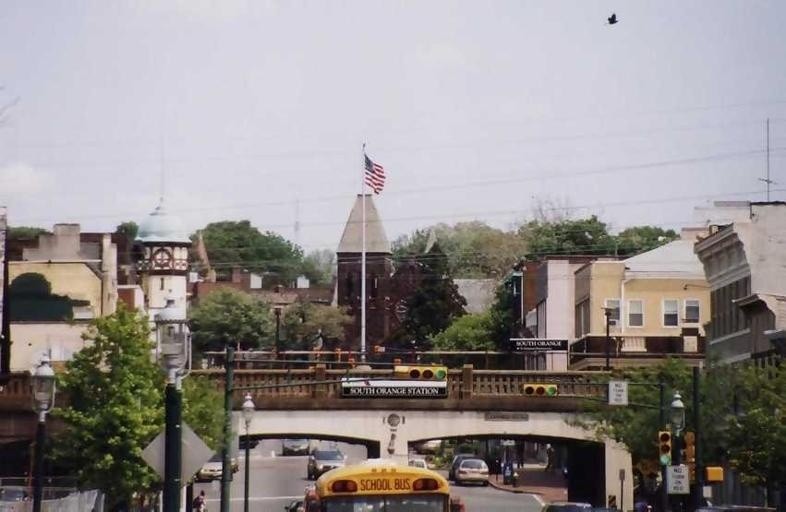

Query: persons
<box><xmin>197</xmin><ymin>490</ymin><xmax>208</xmax><ymax>512</ymax></box>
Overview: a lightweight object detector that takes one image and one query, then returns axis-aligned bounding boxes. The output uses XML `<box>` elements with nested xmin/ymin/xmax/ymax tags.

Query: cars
<box><xmin>238</xmin><ymin>438</ymin><xmax>259</xmax><ymax>451</ymax></box>
<box><xmin>281</xmin><ymin>498</ymin><xmax>304</xmax><ymax>512</ymax></box>
<box><xmin>405</xmin><ymin>458</ymin><xmax>427</xmax><ymax>469</ymax></box>
<box><xmin>454</xmin><ymin>458</ymin><xmax>489</xmax><ymax>488</ymax></box>
<box><xmin>195</xmin><ymin>451</ymin><xmax>233</xmax><ymax>483</ymax></box>
<box><xmin>539</xmin><ymin>500</ymin><xmax>595</xmax><ymax>512</ymax></box>
<box><xmin>230</xmin><ymin>455</ymin><xmax>239</xmax><ymax>474</ymax></box>
<box><xmin>280</xmin><ymin>438</ymin><xmax>310</xmax><ymax>457</ymax></box>
<box><xmin>0</xmin><ymin>484</ymin><xmax>30</xmax><ymax>502</ymax></box>
<box><xmin>301</xmin><ymin>486</ymin><xmax>318</xmax><ymax>512</ymax></box>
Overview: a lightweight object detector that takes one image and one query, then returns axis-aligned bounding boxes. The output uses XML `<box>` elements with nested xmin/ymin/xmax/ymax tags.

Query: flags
<box><xmin>364</xmin><ymin>153</ymin><xmax>385</xmax><ymax>195</ymax></box>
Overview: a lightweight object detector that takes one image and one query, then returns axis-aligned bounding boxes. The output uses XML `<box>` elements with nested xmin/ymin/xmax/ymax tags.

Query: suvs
<box><xmin>305</xmin><ymin>447</ymin><xmax>346</xmax><ymax>482</ymax></box>
<box><xmin>447</xmin><ymin>454</ymin><xmax>480</xmax><ymax>482</ymax></box>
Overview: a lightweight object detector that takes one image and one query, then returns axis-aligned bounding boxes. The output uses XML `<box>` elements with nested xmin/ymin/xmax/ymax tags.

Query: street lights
<box><xmin>600</xmin><ymin>304</ymin><xmax>614</xmax><ymax>372</ymax></box>
<box><xmin>150</xmin><ymin>291</ymin><xmax>191</xmax><ymax>512</ymax></box>
<box><xmin>272</xmin><ymin>305</ymin><xmax>282</xmax><ymax>351</ymax></box>
<box><xmin>238</xmin><ymin>392</ymin><xmax>257</xmax><ymax>512</ymax></box>
<box><xmin>665</xmin><ymin>390</ymin><xmax>687</xmax><ymax>511</ymax></box>
<box><xmin>27</xmin><ymin>347</ymin><xmax>58</xmax><ymax>512</ymax></box>
<box><xmin>173</xmin><ymin>324</ymin><xmax>195</xmax><ymax>512</ymax></box>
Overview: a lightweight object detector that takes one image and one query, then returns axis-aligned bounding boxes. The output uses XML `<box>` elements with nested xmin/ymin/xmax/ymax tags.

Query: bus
<box><xmin>313</xmin><ymin>460</ymin><xmax>467</xmax><ymax>512</ymax></box>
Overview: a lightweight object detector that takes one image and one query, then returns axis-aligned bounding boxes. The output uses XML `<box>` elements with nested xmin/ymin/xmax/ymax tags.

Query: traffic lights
<box><xmin>683</xmin><ymin>431</ymin><xmax>696</xmax><ymax>485</ymax></box>
<box><xmin>393</xmin><ymin>366</ymin><xmax>447</xmax><ymax>381</ymax></box>
<box><xmin>523</xmin><ymin>384</ymin><xmax>557</xmax><ymax>397</ymax></box>
<box><xmin>658</xmin><ymin>430</ymin><xmax>671</xmax><ymax>468</ymax></box>
